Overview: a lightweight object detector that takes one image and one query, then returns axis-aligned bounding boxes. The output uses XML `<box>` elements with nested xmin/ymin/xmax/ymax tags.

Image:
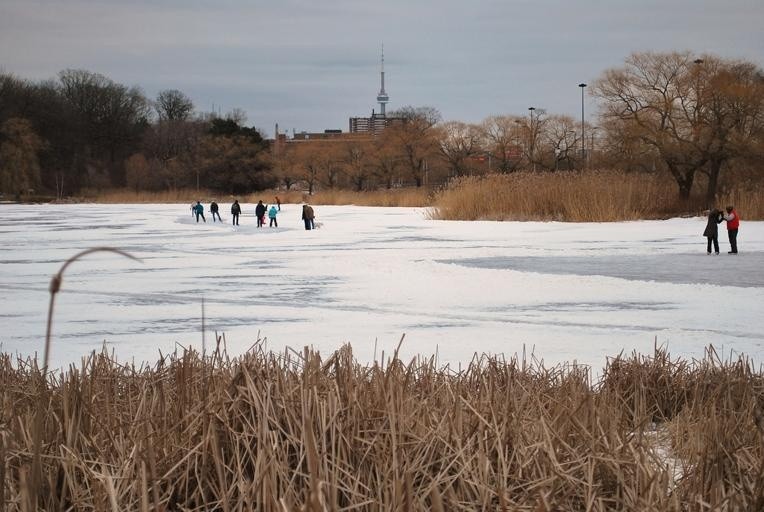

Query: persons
<box><xmin>721</xmin><ymin>205</ymin><xmax>739</xmax><ymax>253</ymax></box>
<box><xmin>302</xmin><ymin>205</ymin><xmax>315</xmax><ymax>230</ymax></box>
<box><xmin>703</xmin><ymin>208</ymin><xmax>724</xmax><ymax>255</ymax></box>
<box><xmin>255</xmin><ymin>197</ymin><xmax>281</xmax><ymax>228</ymax></box>
<box><xmin>190</xmin><ymin>200</ymin><xmax>196</xmax><ymax>217</ymax></box>
<box><xmin>211</xmin><ymin>201</ymin><xmax>222</xmax><ymax>223</ymax></box>
<box><xmin>231</xmin><ymin>200</ymin><xmax>241</xmax><ymax>225</ymax></box>
<box><xmin>195</xmin><ymin>201</ymin><xmax>206</xmax><ymax>223</ymax></box>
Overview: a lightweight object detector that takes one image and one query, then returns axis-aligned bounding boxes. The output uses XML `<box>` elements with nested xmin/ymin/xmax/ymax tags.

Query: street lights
<box><xmin>515</xmin><ymin>120</ymin><xmax>521</xmax><ymax>171</ymax></box>
<box><xmin>528</xmin><ymin>107</ymin><xmax>535</xmax><ymax>171</ymax></box>
<box><xmin>693</xmin><ymin>58</ymin><xmax>703</xmax><ymax>170</ymax></box>
<box><xmin>578</xmin><ymin>83</ymin><xmax>588</xmax><ymax>170</ymax></box>
